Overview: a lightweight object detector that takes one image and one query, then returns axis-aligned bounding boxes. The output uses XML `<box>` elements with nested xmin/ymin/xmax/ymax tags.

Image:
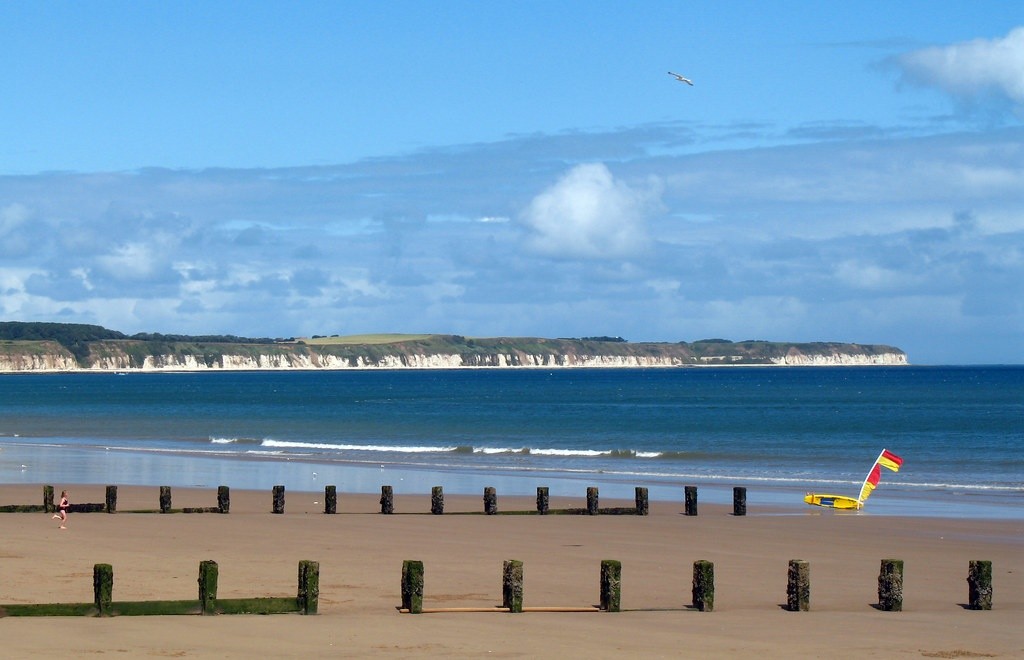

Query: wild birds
<box><xmin>667</xmin><ymin>71</ymin><xmax>694</xmax><ymax>86</ymax></box>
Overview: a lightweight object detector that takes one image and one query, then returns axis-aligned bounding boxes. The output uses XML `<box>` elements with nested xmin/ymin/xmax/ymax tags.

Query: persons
<box><xmin>52</xmin><ymin>489</ymin><xmax>69</xmax><ymax>529</ymax></box>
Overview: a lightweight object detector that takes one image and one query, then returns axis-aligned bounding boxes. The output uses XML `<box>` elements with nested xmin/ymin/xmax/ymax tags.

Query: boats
<box><xmin>804</xmin><ymin>493</ymin><xmax>863</xmax><ymax>509</ymax></box>
